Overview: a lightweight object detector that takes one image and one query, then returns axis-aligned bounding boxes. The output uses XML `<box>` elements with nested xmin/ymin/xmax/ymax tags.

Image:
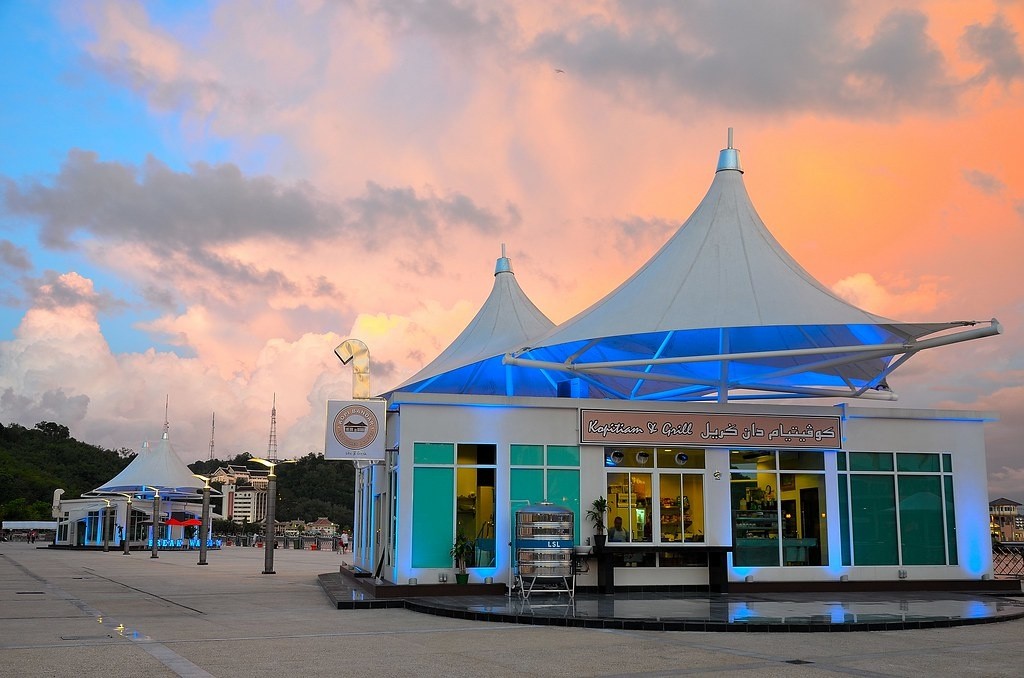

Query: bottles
<box><xmin>739</xmin><ymin>489</ymin><xmax>774</xmax><ymax>510</ymax></box>
<box><xmin>352</xmin><ymin>588</ymin><xmax>364</xmax><ymax>600</ymax></box>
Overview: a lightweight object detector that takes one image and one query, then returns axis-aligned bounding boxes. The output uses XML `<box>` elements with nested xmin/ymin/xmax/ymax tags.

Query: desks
<box><xmin>728</xmin><ymin>536</ymin><xmax>816</xmax><ymax>581</ymax></box>
<box><xmin>594</xmin><ymin>545</ymin><xmax>732</xmax><ymax>593</ymax></box>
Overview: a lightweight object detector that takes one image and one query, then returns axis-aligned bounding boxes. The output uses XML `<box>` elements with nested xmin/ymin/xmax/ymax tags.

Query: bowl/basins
<box><xmin>576</xmin><ymin>545</ymin><xmax>592</xmax><ymax>555</ymax></box>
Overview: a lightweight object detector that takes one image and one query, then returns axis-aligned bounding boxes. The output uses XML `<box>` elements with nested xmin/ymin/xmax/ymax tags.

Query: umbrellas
<box><xmin>138</xmin><ymin>519</ymin><xmax>164</xmax><ymax>525</ymax></box>
<box><xmin>166</xmin><ymin>518</ymin><xmax>181</xmax><ymax>525</ymax></box>
<box><xmin>182</xmin><ymin>519</ymin><xmax>202</xmax><ymax>526</ymax></box>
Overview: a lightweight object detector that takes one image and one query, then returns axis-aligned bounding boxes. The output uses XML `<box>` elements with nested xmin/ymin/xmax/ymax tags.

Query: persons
<box><xmin>338</xmin><ymin>538</ymin><xmax>346</xmax><ymax>552</ymax></box>
<box><xmin>644</xmin><ymin>512</ymin><xmax>663</xmax><ymax>539</ymax></box>
<box><xmin>251</xmin><ymin>532</ymin><xmax>258</xmax><ymax>546</ymax></box>
<box><xmin>608</xmin><ymin>517</ymin><xmax>628</xmax><ymax>541</ymax></box>
<box><xmin>341</xmin><ymin>530</ymin><xmax>349</xmax><ymax>549</ymax></box>
<box><xmin>26</xmin><ymin>530</ymin><xmax>37</xmax><ymax>544</ymax></box>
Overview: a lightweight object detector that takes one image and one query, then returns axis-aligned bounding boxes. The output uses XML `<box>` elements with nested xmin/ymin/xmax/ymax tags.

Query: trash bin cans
<box><xmin>242</xmin><ymin>538</ymin><xmax>247</xmax><ymax>547</ymax></box>
<box><xmin>293</xmin><ymin>539</ymin><xmax>299</xmax><ymax>549</ymax></box>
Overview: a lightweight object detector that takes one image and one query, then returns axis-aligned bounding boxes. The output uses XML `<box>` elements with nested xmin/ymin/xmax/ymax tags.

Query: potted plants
<box><xmin>450</xmin><ymin>534</ymin><xmax>479</xmax><ymax>585</ymax></box>
<box><xmin>586</xmin><ymin>496</ymin><xmax>612</xmax><ymax>545</ymax></box>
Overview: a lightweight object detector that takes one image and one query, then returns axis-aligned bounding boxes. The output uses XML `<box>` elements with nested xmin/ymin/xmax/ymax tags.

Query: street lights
<box><xmin>191</xmin><ymin>473</ymin><xmax>224</xmax><ymax>565</ymax></box>
<box><xmin>100</xmin><ymin>498</ymin><xmax>120</xmax><ymax>552</ymax></box>
<box><xmin>247</xmin><ymin>457</ymin><xmax>300</xmax><ymax>575</ymax></box>
<box><xmin>146</xmin><ymin>486</ymin><xmax>173</xmax><ymax>559</ymax></box>
<box><xmin>116</xmin><ymin>493</ymin><xmax>142</xmax><ymax>555</ymax></box>
